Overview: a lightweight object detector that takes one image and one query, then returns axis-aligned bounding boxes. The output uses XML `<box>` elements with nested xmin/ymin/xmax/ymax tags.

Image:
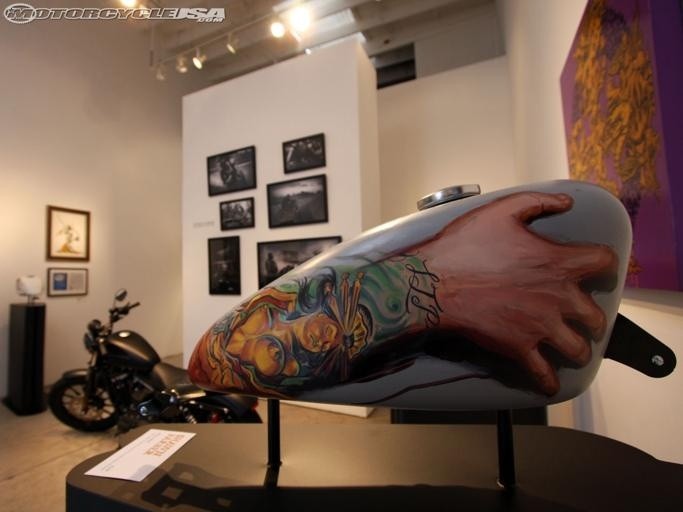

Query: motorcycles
<box><xmin>47</xmin><ymin>287</ymin><xmax>262</xmax><ymax>434</ymax></box>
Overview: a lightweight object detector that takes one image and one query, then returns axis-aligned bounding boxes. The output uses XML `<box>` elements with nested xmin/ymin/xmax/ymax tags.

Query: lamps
<box><xmin>154</xmin><ymin>30</ymin><xmax>238</xmax><ymax>80</ymax></box>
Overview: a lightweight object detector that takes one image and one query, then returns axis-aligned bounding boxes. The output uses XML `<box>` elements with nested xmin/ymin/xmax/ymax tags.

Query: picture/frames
<box><xmin>46</xmin><ymin>205</ymin><xmax>89</xmax><ymax>296</ymax></box>
<box><xmin>206</xmin><ymin>133</ymin><xmax>341</xmax><ymax>295</ymax></box>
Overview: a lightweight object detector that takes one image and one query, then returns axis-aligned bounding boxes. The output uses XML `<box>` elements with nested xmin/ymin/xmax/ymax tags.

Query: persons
<box><xmin>263</xmin><ymin>253</ymin><xmax>278</xmax><ymax>275</ymax></box>
<box><xmin>186</xmin><ymin>192</ymin><xmax>618</xmax><ymax>416</ymax></box>
<box><xmin>196</xmin><ymin>265</ymin><xmax>371</xmax><ymax>396</ymax></box>
